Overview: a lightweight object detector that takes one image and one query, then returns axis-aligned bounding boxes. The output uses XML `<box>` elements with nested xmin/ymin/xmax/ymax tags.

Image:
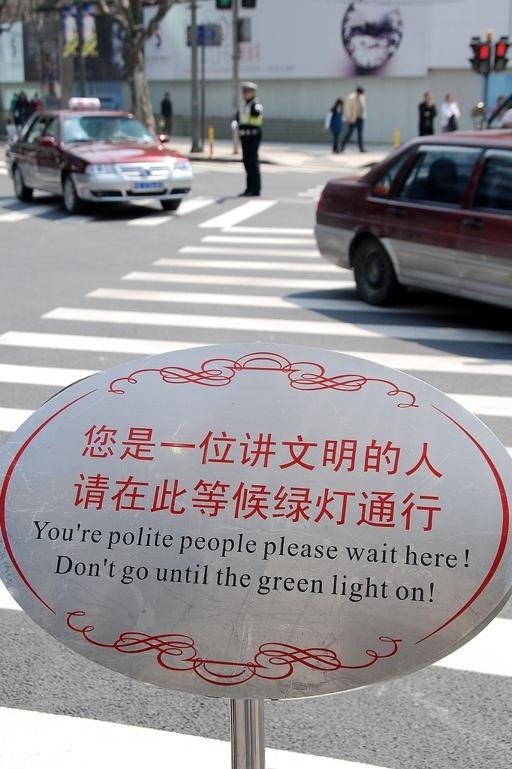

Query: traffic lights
<box><xmin>241</xmin><ymin>0</ymin><xmax>258</xmax><ymax>9</ymax></box>
<box><xmin>477</xmin><ymin>35</ymin><xmax>491</xmax><ymax>74</ymax></box>
<box><xmin>494</xmin><ymin>35</ymin><xmax>511</xmax><ymax>71</ymax></box>
<box><xmin>215</xmin><ymin>0</ymin><xmax>232</xmax><ymax>9</ymax></box>
<box><xmin>468</xmin><ymin>35</ymin><xmax>480</xmax><ymax>70</ymax></box>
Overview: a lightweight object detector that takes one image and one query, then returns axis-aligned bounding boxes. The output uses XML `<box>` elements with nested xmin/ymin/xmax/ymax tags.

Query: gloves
<box><xmin>231</xmin><ymin>120</ymin><xmax>238</xmax><ymax>130</ymax></box>
<box><xmin>255</xmin><ymin>103</ymin><xmax>264</xmax><ymax>112</ymax></box>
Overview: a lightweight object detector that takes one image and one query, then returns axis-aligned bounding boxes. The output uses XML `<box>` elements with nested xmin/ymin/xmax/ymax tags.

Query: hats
<box><xmin>241</xmin><ymin>81</ymin><xmax>257</xmax><ymax>91</ymax></box>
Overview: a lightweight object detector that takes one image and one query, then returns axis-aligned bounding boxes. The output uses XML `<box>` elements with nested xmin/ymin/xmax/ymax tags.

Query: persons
<box><xmin>159</xmin><ymin>91</ymin><xmax>174</xmax><ymax>136</ymax></box>
<box><xmin>230</xmin><ymin>81</ymin><xmax>265</xmax><ymax>197</ymax></box>
<box><xmin>15</xmin><ymin>90</ymin><xmax>32</xmax><ymax>133</ymax></box>
<box><xmin>342</xmin><ymin>86</ymin><xmax>368</xmax><ymax>152</ymax></box>
<box><xmin>472</xmin><ymin>102</ymin><xmax>485</xmax><ymax>130</ymax></box>
<box><xmin>439</xmin><ymin>91</ymin><xmax>461</xmax><ymax>132</ymax></box>
<box><xmin>417</xmin><ymin>89</ymin><xmax>437</xmax><ymax>136</ymax></box>
<box><xmin>29</xmin><ymin>91</ymin><xmax>42</xmax><ymax>112</ymax></box>
<box><xmin>9</xmin><ymin>90</ymin><xmax>19</xmax><ymax>121</ymax></box>
<box><xmin>323</xmin><ymin>98</ymin><xmax>346</xmax><ymax>153</ymax></box>
<box><xmin>492</xmin><ymin>94</ymin><xmax>508</xmax><ymax>130</ymax></box>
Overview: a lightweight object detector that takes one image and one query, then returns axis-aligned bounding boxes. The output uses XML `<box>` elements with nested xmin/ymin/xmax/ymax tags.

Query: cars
<box><xmin>310</xmin><ymin>123</ymin><xmax>512</xmax><ymax>313</ymax></box>
<box><xmin>3</xmin><ymin>95</ymin><xmax>197</xmax><ymax>214</ymax></box>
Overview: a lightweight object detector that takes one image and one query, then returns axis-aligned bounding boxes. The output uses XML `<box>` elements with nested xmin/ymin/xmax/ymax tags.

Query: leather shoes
<box><xmin>242</xmin><ymin>190</ymin><xmax>259</xmax><ymax>196</ymax></box>
